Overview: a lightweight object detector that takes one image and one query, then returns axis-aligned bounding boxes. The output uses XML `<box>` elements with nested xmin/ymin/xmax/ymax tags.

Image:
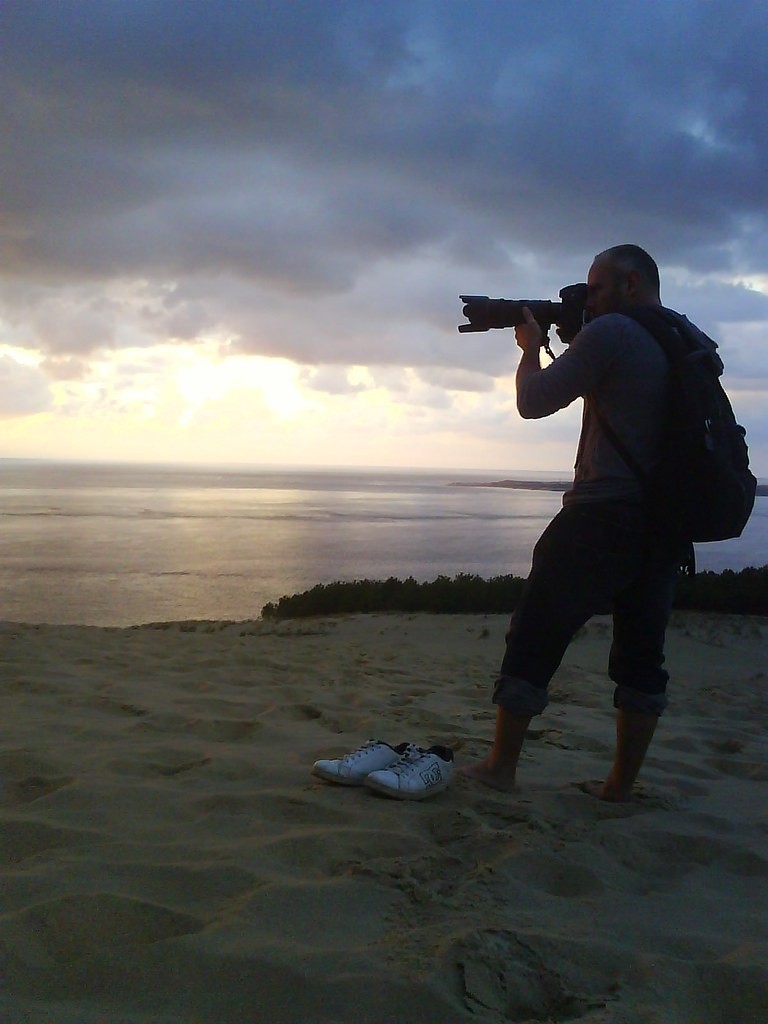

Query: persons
<box><xmin>451</xmin><ymin>244</ymin><xmax>693</xmax><ymax>802</ymax></box>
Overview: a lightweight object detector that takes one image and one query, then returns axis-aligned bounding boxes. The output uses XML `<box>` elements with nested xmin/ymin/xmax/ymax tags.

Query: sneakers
<box><xmin>364</xmin><ymin>742</ymin><xmax>458</xmax><ymax>801</ymax></box>
<box><xmin>310</xmin><ymin>738</ymin><xmax>416</xmax><ymax>786</ymax></box>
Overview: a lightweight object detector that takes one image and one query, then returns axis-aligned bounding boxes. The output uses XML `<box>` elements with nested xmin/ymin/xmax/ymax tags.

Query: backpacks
<box><xmin>596</xmin><ymin>307</ymin><xmax>758</xmax><ymax>541</ymax></box>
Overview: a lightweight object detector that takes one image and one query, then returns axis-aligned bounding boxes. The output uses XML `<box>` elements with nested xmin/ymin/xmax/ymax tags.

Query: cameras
<box><xmin>458</xmin><ymin>283</ymin><xmax>590</xmax><ymax>343</ymax></box>
